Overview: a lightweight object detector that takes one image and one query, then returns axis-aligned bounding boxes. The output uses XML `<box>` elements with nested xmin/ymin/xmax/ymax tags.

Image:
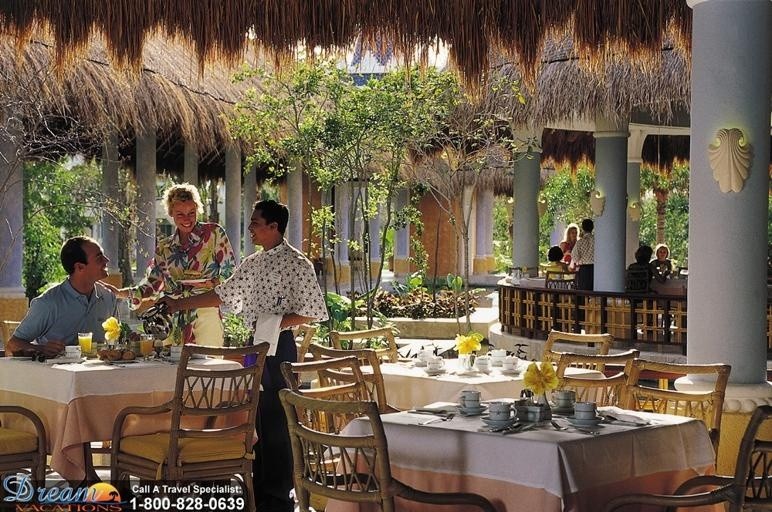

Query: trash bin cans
<box><xmin>314</xmin><ymin>257</ymin><xmax>329</xmax><ymax>275</ymax></box>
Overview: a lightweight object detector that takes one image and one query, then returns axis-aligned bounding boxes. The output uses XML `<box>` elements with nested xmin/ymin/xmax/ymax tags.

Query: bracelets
<box><xmin>178</xmin><ymin>296</ymin><xmax>182</xmax><ymax>313</ymax></box>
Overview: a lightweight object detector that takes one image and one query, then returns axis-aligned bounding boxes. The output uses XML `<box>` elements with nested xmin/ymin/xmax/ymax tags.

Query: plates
<box><xmin>502</xmin><ymin>369</ymin><xmax>521</xmax><ymax>375</ymax></box>
<box><xmin>549</xmin><ymin>406</ymin><xmax>575</xmax><ymax>413</ymax></box>
<box><xmin>165</xmin><ymin>356</ymin><xmax>192</xmax><ymax>362</ymax></box>
<box><xmin>415</xmin><ymin>361</ymin><xmax>427</xmax><ymax>367</ymax></box>
<box><xmin>178</xmin><ymin>279</ymin><xmax>209</xmax><ymax>283</ymax></box>
<box><xmin>481</xmin><ymin>417</ymin><xmax>520</xmax><ymax>428</ymax></box>
<box><xmin>102</xmin><ymin>358</ymin><xmax>138</xmax><ymax>364</ymax></box>
<box><xmin>565</xmin><ymin>415</ymin><xmax>603</xmax><ymax>427</ymax></box>
<box><xmin>424</xmin><ymin>369</ymin><xmax>447</xmax><ymax>376</ymax></box>
<box><xmin>459</xmin><ymin>406</ymin><xmax>487</xmax><ymax>416</ymax></box>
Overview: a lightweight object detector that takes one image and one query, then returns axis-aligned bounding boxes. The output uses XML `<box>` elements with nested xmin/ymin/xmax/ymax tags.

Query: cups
<box><xmin>551</xmin><ymin>389</ymin><xmax>576</xmax><ymax>407</ymax></box>
<box><xmin>78</xmin><ymin>332</ymin><xmax>92</xmax><ymax>357</ymax></box>
<box><xmin>171</xmin><ymin>345</ymin><xmax>184</xmax><ymax>359</ymax></box>
<box><xmin>460</xmin><ymin>390</ymin><xmax>481</xmax><ymax>407</ymax></box>
<box><xmin>572</xmin><ymin>401</ymin><xmax>598</xmax><ymax>421</ymax></box>
<box><xmin>487</xmin><ymin>350</ymin><xmax>506</xmax><ymax>364</ymax></box>
<box><xmin>128</xmin><ymin>341</ymin><xmax>139</xmax><ymax>357</ymax></box>
<box><xmin>65</xmin><ymin>346</ymin><xmax>81</xmax><ymax>358</ymax></box>
<box><xmin>427</xmin><ymin>356</ymin><xmax>446</xmax><ymax>370</ymax></box>
<box><xmin>504</xmin><ymin>357</ymin><xmax>518</xmax><ymax>371</ymax></box>
<box><xmin>489</xmin><ymin>401</ymin><xmax>517</xmax><ymax>422</ymax></box>
<box><xmin>416</xmin><ymin>350</ymin><xmax>436</xmax><ymax>362</ymax></box>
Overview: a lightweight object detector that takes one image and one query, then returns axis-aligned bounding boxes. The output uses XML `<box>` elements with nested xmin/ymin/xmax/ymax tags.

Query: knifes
<box><xmin>408</xmin><ymin>411</ymin><xmax>434</xmax><ymax>416</ymax></box>
<box><xmin>488</xmin><ymin>423</ymin><xmax>538</xmax><ymax>435</ymax></box>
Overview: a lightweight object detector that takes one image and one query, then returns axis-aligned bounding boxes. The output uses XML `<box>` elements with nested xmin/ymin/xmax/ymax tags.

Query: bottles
<box><xmin>154</xmin><ymin>337</ymin><xmax>163</xmax><ymax>359</ymax></box>
<box><xmin>520</xmin><ymin>389</ymin><xmax>534</xmax><ymax>403</ymax></box>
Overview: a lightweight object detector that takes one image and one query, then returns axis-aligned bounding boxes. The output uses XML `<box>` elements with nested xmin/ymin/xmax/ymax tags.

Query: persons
<box><xmin>156</xmin><ymin>199</ymin><xmax>330</xmax><ymax>512</ymax></box>
<box><xmin>627</xmin><ymin>246</ymin><xmax>670</xmax><ymax>332</ymax></box>
<box><xmin>572</xmin><ymin>218</ymin><xmax>594</xmax><ymax>321</ymax></box>
<box><xmin>4</xmin><ymin>235</ymin><xmax>121</xmax><ymax>357</ymax></box>
<box><xmin>98</xmin><ymin>181</ymin><xmax>237</xmax><ymax>360</ymax></box>
<box><xmin>559</xmin><ymin>226</ymin><xmax>577</xmax><ymax>264</ymax></box>
<box><xmin>650</xmin><ymin>244</ymin><xmax>672</xmax><ymax>276</ymax></box>
<box><xmin>544</xmin><ymin>246</ymin><xmax>570</xmax><ymax>303</ymax></box>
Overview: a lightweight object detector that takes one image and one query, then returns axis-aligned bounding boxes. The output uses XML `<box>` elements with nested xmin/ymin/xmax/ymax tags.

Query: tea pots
<box><xmin>136</xmin><ymin>302</ymin><xmax>173</xmax><ymax>342</ymax></box>
<box><xmin>475</xmin><ymin>356</ymin><xmax>493</xmax><ymax>371</ymax></box>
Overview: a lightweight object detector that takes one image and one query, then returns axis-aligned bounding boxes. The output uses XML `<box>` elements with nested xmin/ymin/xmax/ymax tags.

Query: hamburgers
<box><xmin>183</xmin><ymin>270</ymin><xmax>200</xmax><ymax>280</ymax></box>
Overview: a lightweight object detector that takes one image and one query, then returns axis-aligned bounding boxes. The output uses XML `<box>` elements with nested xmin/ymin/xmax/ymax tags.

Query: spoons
<box><xmin>416</xmin><ymin>409</ymin><xmax>446</xmax><ymax>414</ymax></box>
<box><xmin>606</xmin><ymin>415</ymin><xmax>646</xmax><ymax>425</ymax></box>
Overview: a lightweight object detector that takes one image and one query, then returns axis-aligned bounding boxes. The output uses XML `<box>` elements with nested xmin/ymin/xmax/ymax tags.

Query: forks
<box><xmin>54</xmin><ymin>360</ymin><xmax>85</xmax><ymax>365</ymax></box>
<box><xmin>550</xmin><ymin>421</ymin><xmax>600</xmax><ymax>435</ymax></box>
<box><xmin>418</xmin><ymin>412</ymin><xmax>455</xmax><ymax>425</ymax></box>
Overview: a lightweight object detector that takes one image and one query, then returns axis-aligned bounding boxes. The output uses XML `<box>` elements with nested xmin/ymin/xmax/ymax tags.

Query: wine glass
<box><xmin>139</xmin><ymin>334</ymin><xmax>154</xmax><ymax>363</ymax></box>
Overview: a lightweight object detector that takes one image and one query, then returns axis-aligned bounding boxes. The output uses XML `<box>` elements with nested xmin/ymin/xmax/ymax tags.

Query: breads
<box><xmin>98</xmin><ymin>349</ymin><xmax>139</xmax><ymax>362</ymax></box>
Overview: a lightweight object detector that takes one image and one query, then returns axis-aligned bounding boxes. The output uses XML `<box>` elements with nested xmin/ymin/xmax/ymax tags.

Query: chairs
<box><xmin>0</xmin><ymin>318</ymin><xmax>772</xmax><ymax>512</ymax></box>
<box><xmin>510</xmin><ymin>264</ymin><xmax>688</xmax><ymax>294</ymax></box>
